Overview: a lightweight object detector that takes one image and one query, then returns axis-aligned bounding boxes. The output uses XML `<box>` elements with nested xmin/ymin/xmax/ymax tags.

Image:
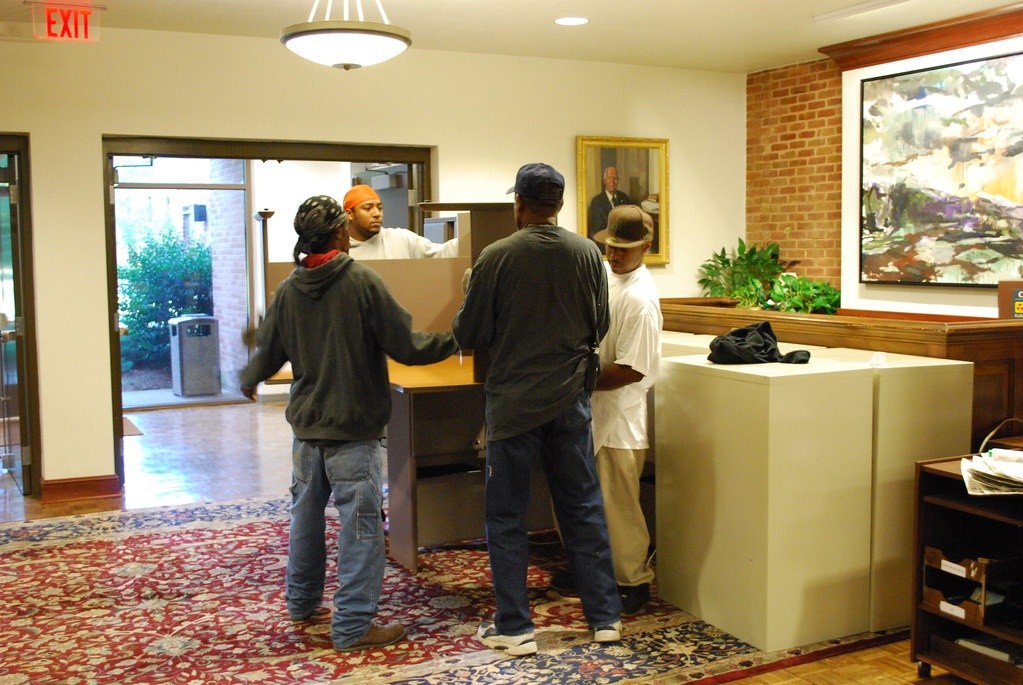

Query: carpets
<box><xmin>0</xmin><ymin>484</ymin><xmax>907</xmax><ymax>684</ymax></box>
<box><xmin>1</xmin><ymin>414</ymin><xmax>144</xmax><ymax>446</ymax></box>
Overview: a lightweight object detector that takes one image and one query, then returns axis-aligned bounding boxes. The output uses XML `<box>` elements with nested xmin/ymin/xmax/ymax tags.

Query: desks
<box><xmin>384</xmin><ymin>367</ymin><xmax>649</xmax><ymax>571</ymax></box>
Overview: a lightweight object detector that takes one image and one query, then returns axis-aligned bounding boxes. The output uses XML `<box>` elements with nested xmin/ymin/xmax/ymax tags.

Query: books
<box><xmin>960</xmin><ymin>448</ymin><xmax>1023</xmax><ymax>497</ymax></box>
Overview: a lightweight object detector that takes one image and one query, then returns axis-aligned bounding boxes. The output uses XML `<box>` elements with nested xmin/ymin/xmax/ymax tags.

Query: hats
<box><xmin>594</xmin><ymin>204</ymin><xmax>653</xmax><ymax>248</ymax></box>
<box><xmin>506</xmin><ymin>163</ymin><xmax>565</xmax><ymax>206</ymax></box>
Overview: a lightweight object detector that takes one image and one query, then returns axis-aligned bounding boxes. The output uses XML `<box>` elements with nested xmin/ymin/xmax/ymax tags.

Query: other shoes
<box><xmin>551</xmin><ymin>575</ymin><xmax>576</xmax><ymax>592</ymax></box>
<box><xmin>292</xmin><ymin>614</ymin><xmax>310</xmax><ymax>625</ymax></box>
<box><xmin>334</xmin><ymin>623</ymin><xmax>406</xmax><ymax>651</ymax></box>
<box><xmin>619</xmin><ymin>583</ymin><xmax>650</xmax><ymax>614</ymax></box>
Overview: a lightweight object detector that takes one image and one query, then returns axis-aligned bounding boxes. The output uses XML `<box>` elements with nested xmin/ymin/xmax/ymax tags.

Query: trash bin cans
<box><xmin>168</xmin><ymin>313</ymin><xmax>221</xmax><ymax>398</ymax></box>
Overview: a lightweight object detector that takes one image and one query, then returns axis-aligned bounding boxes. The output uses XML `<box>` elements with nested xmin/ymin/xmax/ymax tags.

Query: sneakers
<box><xmin>594</xmin><ymin>620</ymin><xmax>622</xmax><ymax>642</ymax></box>
<box><xmin>477</xmin><ymin>622</ymin><xmax>537</xmax><ymax>655</ymax></box>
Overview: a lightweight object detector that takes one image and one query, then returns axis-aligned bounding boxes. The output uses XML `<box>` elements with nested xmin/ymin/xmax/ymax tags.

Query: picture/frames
<box><xmin>858</xmin><ymin>54</ymin><xmax>1023</xmax><ymax>286</ymax></box>
<box><xmin>575</xmin><ymin>133</ymin><xmax>671</xmax><ymax>268</ymax></box>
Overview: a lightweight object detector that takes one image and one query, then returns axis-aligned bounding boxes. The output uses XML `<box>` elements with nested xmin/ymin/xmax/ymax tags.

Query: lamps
<box><xmin>280</xmin><ymin>1</ymin><xmax>412</xmax><ymax>69</ymax></box>
<box><xmin>553</xmin><ymin>16</ymin><xmax>590</xmax><ymax>24</ymax></box>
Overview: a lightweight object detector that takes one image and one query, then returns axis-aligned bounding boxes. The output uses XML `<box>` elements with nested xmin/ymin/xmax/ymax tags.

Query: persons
<box><xmin>549</xmin><ymin>204</ymin><xmax>665</xmax><ymax>620</ymax></box>
<box><xmin>341</xmin><ymin>183</ymin><xmax>461</xmax><ymax>260</ymax></box>
<box><xmin>449</xmin><ymin>160</ymin><xmax>626</xmax><ymax>658</ymax></box>
<box><xmin>588</xmin><ymin>165</ymin><xmax>643</xmax><ymax>256</ymax></box>
<box><xmin>236</xmin><ymin>194</ymin><xmax>461</xmax><ymax>653</ymax></box>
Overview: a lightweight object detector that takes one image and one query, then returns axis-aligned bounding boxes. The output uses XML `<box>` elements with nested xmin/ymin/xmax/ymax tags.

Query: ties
<box><xmin>611</xmin><ymin>195</ymin><xmax>616</xmax><ymax>208</ymax></box>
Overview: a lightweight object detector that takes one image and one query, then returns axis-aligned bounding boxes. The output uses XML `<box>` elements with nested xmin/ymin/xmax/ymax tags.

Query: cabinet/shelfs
<box><xmin>910</xmin><ymin>452</ymin><xmax>1023</xmax><ymax>685</ymax></box>
